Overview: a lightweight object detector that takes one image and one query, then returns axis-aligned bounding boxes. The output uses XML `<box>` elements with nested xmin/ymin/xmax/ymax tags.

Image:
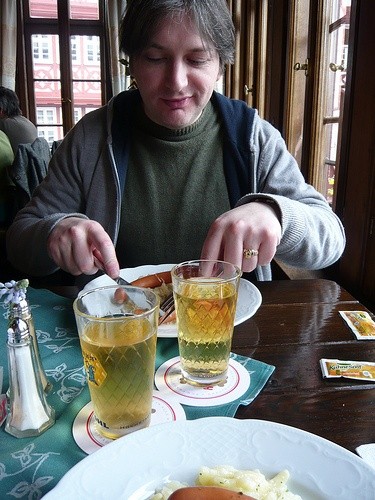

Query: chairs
<box><xmin>0</xmin><ymin>136</ymin><xmax>54</xmax><ymax>235</ymax></box>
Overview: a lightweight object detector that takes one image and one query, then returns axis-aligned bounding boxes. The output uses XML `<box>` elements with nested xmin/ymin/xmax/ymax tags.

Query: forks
<box><xmin>158</xmin><ymin>265</ymin><xmax>224</xmax><ymax>326</ymax></box>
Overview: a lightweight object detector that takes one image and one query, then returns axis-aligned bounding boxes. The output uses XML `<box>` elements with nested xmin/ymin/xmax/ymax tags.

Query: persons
<box><xmin>0</xmin><ymin>85</ymin><xmax>37</xmax><ymax>170</ymax></box>
<box><xmin>5</xmin><ymin>0</ymin><xmax>346</xmax><ymax>280</ymax></box>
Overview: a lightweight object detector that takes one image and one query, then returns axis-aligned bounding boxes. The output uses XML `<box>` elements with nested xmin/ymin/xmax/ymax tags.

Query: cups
<box><xmin>171</xmin><ymin>260</ymin><xmax>241</xmax><ymax>384</ymax></box>
<box><xmin>73</xmin><ymin>285</ymin><xmax>160</xmax><ymax>438</ymax></box>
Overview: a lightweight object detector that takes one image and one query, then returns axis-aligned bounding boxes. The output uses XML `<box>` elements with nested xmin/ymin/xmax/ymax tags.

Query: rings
<box><xmin>243</xmin><ymin>249</ymin><xmax>259</xmax><ymax>257</ymax></box>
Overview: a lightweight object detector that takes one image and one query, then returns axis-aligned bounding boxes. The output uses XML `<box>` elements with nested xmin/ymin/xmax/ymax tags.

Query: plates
<box><xmin>77</xmin><ymin>264</ymin><xmax>262</xmax><ymax>338</ymax></box>
<box><xmin>39</xmin><ymin>417</ymin><xmax>375</xmax><ymax>500</ymax></box>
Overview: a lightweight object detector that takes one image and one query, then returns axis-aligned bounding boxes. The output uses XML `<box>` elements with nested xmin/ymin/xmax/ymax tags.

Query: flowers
<box><xmin>0</xmin><ymin>279</ymin><xmax>30</xmax><ymax>328</ymax></box>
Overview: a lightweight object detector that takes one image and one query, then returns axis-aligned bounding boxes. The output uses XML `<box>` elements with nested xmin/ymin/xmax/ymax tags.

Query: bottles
<box><xmin>5</xmin><ymin>318</ymin><xmax>56</xmax><ymax>439</ymax></box>
<box><xmin>6</xmin><ymin>300</ymin><xmax>53</xmax><ymax>400</ymax></box>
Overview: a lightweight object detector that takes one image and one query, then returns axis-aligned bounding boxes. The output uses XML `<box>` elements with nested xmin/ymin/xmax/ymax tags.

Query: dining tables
<box><xmin>0</xmin><ymin>278</ymin><xmax>375</xmax><ymax>500</ymax></box>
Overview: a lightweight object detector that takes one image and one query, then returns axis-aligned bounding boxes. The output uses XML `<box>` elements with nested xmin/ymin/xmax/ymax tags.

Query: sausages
<box><xmin>113</xmin><ymin>271</ymin><xmax>171</xmax><ymax>305</ymax></box>
<box><xmin>167</xmin><ymin>486</ymin><xmax>259</xmax><ymax>500</ymax></box>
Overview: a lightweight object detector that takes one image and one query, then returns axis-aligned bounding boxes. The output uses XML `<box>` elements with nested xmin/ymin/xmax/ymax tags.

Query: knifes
<box><xmin>93</xmin><ymin>255</ymin><xmax>166</xmax><ymax>316</ymax></box>
